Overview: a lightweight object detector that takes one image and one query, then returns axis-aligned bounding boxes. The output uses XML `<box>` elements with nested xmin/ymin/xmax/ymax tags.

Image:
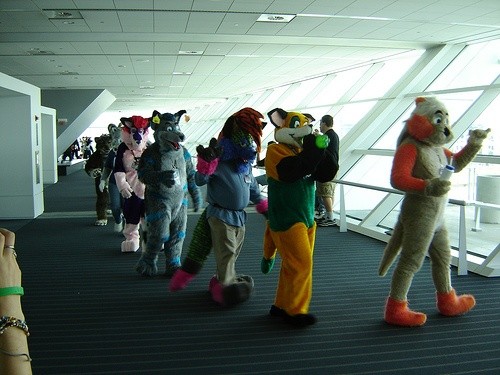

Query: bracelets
<box><xmin>0</xmin><ymin>349</ymin><xmax>32</xmax><ymax>361</ymax></box>
<box><xmin>0</xmin><ymin>317</ymin><xmax>30</xmax><ymax>336</ymax></box>
<box><xmin>0</xmin><ymin>287</ymin><xmax>24</xmax><ymax>296</ymax></box>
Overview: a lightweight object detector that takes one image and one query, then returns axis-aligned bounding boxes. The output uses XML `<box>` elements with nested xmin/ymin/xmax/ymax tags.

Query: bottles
<box><xmin>438</xmin><ymin>164</ymin><xmax>455</xmax><ymax>180</ymax></box>
<box><xmin>300</xmin><ymin>133</ymin><xmax>330</xmax><ymax>148</ymax></box>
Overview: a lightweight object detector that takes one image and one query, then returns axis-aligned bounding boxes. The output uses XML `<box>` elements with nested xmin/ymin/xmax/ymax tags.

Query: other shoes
<box><xmin>317</xmin><ymin>216</ymin><xmax>337</xmax><ymax>227</ymax></box>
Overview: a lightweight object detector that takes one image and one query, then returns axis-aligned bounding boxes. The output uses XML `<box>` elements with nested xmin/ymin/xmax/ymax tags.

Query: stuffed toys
<box><xmin>376</xmin><ymin>97</ymin><xmax>491</xmax><ymax>326</ymax></box>
<box><xmin>136</xmin><ymin>110</ymin><xmax>202</xmax><ymax>276</ymax></box>
<box><xmin>170</xmin><ymin>107</ymin><xmax>268</xmax><ymax>304</ymax></box>
<box><xmin>84</xmin><ymin>134</ymin><xmax>113</xmax><ymax>224</ymax></box>
<box><xmin>260</xmin><ymin>108</ymin><xmax>340</xmax><ymax>324</ymax></box>
<box><xmin>99</xmin><ymin>124</ymin><xmax>123</xmax><ymax>231</ymax></box>
<box><xmin>113</xmin><ymin>115</ymin><xmax>165</xmax><ymax>252</ymax></box>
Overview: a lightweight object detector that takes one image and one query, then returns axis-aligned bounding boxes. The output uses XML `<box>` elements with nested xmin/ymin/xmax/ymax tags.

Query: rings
<box><xmin>13</xmin><ymin>253</ymin><xmax>17</xmax><ymax>257</ymax></box>
<box><xmin>4</xmin><ymin>245</ymin><xmax>14</xmax><ymax>249</ymax></box>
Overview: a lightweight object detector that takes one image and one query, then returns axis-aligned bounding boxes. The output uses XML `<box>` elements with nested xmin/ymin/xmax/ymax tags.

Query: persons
<box><xmin>0</xmin><ymin>228</ymin><xmax>32</xmax><ymax>375</ymax></box>
<box><xmin>62</xmin><ymin>139</ymin><xmax>94</xmax><ymax>161</ymax></box>
<box><xmin>314</xmin><ymin>115</ymin><xmax>339</xmax><ymax>226</ymax></box>
